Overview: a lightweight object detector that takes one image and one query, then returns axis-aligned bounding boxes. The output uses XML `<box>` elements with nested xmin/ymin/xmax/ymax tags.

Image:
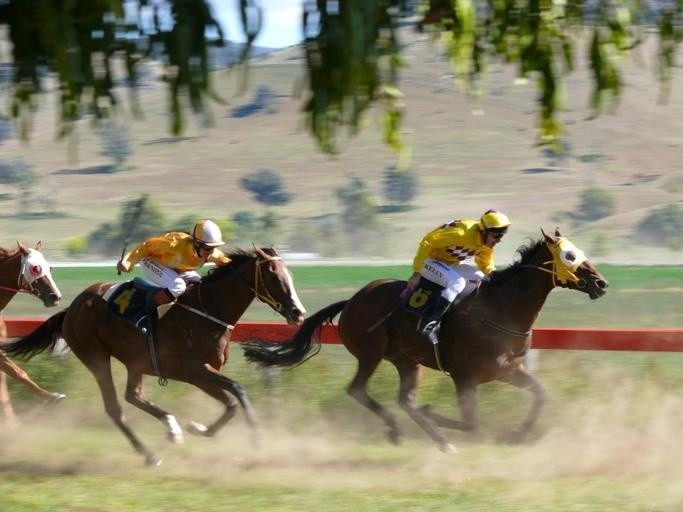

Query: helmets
<box><xmin>478</xmin><ymin>208</ymin><xmax>511</xmax><ymax>231</ymax></box>
<box><xmin>192</xmin><ymin>219</ymin><xmax>225</xmax><ymax>247</ymax></box>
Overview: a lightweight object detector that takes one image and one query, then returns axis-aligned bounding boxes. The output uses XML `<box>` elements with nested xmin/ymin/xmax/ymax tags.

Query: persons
<box><xmin>405</xmin><ymin>209</ymin><xmax>511</xmax><ymax>346</ymax></box>
<box><xmin>115</xmin><ymin>218</ymin><xmax>231</xmax><ymax>335</ymax></box>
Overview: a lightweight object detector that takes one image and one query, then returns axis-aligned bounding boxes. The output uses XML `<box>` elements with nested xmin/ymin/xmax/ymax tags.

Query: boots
<box><xmin>416</xmin><ymin>296</ymin><xmax>452</xmax><ymax>339</ymax></box>
<box><xmin>129</xmin><ymin>288</ymin><xmax>177</xmax><ymax>328</ymax></box>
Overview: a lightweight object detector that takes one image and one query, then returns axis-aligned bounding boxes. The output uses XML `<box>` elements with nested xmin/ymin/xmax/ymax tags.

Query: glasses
<box><xmin>491</xmin><ymin>230</ymin><xmax>505</xmax><ymax>238</ymax></box>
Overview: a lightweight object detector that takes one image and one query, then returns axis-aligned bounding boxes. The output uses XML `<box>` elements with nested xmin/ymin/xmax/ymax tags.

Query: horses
<box><xmin>0</xmin><ymin>239</ymin><xmax>68</xmax><ymax>431</ymax></box>
<box><xmin>242</xmin><ymin>225</ymin><xmax>610</xmax><ymax>455</ymax></box>
<box><xmin>0</xmin><ymin>240</ymin><xmax>308</xmax><ymax>469</ymax></box>
